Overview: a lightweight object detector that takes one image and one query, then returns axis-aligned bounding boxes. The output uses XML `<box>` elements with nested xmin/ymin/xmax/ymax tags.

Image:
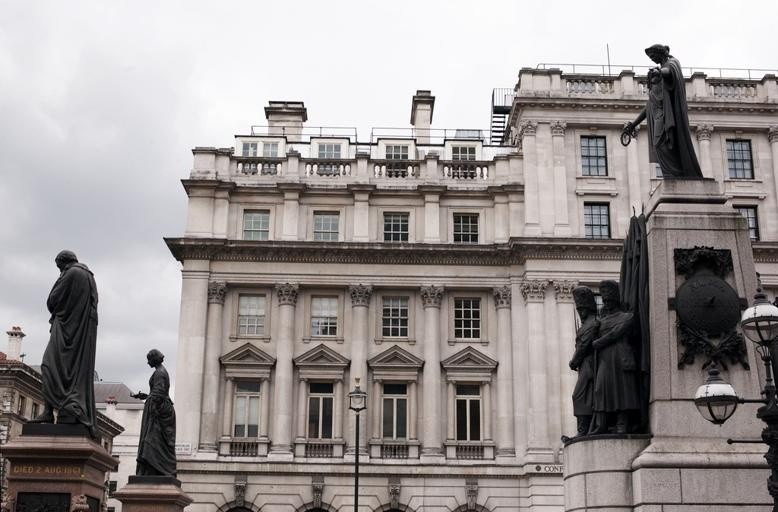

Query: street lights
<box><xmin>694</xmin><ymin>286</ymin><xmax>778</xmax><ymax>512</ymax></box>
<box><xmin>346</xmin><ymin>376</ymin><xmax>368</xmax><ymax>512</ymax></box>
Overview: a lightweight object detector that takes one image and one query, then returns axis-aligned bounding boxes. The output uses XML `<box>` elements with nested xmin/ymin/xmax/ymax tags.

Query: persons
<box><xmin>28</xmin><ymin>249</ymin><xmax>100</xmax><ymax>437</ymax></box>
<box><xmin>588</xmin><ymin>280</ymin><xmax>637</xmax><ymax>436</ymax></box>
<box><xmin>560</xmin><ymin>287</ymin><xmax>600</xmax><ymax>444</ymax></box>
<box><xmin>129</xmin><ymin>349</ymin><xmax>178</xmax><ymax>477</ymax></box>
<box><xmin>624</xmin><ymin>44</ymin><xmax>703</xmax><ymax>179</ymax></box>
